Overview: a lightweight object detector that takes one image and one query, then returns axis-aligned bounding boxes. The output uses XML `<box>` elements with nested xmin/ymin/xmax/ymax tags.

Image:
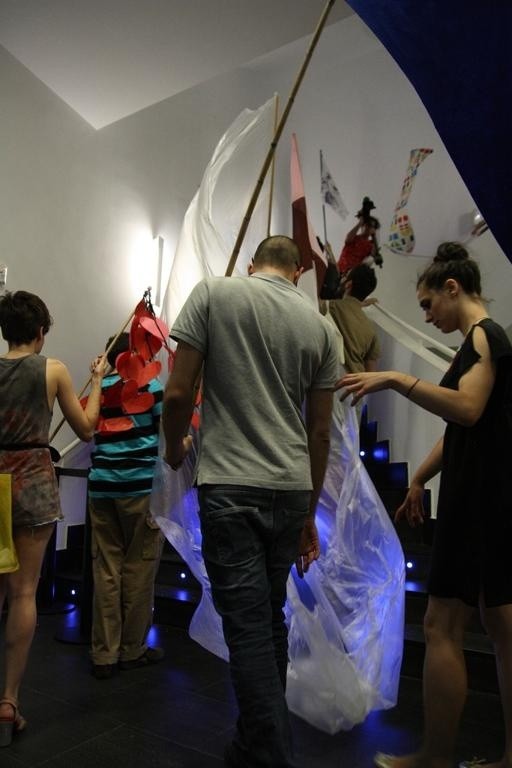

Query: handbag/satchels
<box><xmin>1</xmin><ymin>476</ymin><xmax>19</xmax><ymax>573</ymax></box>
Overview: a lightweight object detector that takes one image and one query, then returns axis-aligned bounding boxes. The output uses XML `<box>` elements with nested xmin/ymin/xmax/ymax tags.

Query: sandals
<box><xmin>374</xmin><ymin>752</ymin><xmax>396</xmax><ymax>768</ymax></box>
<box><xmin>459</xmin><ymin>757</ymin><xmax>486</xmax><ymax>768</ymax></box>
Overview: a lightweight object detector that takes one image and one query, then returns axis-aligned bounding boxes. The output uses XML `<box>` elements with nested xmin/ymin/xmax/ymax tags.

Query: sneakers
<box><xmin>92</xmin><ymin>647</ymin><xmax>166</xmax><ymax>679</ymax></box>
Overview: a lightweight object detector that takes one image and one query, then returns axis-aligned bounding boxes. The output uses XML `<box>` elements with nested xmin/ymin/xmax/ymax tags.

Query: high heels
<box><xmin>0</xmin><ymin>696</ymin><xmax>26</xmax><ymax>747</ymax></box>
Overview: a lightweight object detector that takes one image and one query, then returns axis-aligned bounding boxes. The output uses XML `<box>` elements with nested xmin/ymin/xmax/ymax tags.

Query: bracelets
<box><xmin>406</xmin><ymin>379</ymin><xmax>420</xmax><ymax>397</ymax></box>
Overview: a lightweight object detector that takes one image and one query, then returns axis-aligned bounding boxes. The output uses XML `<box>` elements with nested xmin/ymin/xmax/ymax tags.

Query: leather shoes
<box><xmin>223</xmin><ymin>749</ymin><xmax>253</xmax><ymax>768</ymax></box>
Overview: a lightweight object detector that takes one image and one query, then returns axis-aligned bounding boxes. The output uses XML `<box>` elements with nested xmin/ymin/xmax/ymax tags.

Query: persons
<box><xmin>331</xmin><ymin>240</ymin><xmax>512</xmax><ymax>767</ymax></box>
<box><xmin>325</xmin><ymin>262</ymin><xmax>380</xmax><ymax>427</ymax></box>
<box><xmin>85</xmin><ymin>332</ymin><xmax>166</xmax><ymax>678</ymax></box>
<box><xmin>337</xmin><ymin>213</ymin><xmax>380</xmax><ymax>276</ymax></box>
<box><xmin>0</xmin><ymin>289</ymin><xmax>113</xmax><ymax>748</ymax></box>
<box><xmin>155</xmin><ymin>234</ymin><xmax>346</xmax><ymax>768</ymax></box>
